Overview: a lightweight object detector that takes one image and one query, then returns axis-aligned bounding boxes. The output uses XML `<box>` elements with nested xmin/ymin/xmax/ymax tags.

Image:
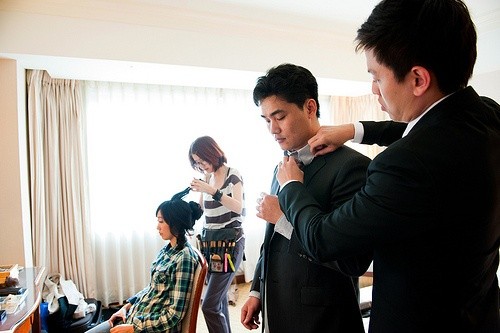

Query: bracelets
<box><xmin>213</xmin><ymin>189</ymin><xmax>223</xmax><ymax>201</ymax></box>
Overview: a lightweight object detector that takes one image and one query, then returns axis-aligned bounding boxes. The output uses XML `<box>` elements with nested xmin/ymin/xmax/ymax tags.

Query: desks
<box><xmin>0</xmin><ymin>266</ymin><xmax>45</xmax><ymax>333</ymax></box>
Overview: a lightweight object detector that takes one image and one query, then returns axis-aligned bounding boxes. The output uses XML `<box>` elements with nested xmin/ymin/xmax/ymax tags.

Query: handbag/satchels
<box><xmin>47</xmin><ymin>294</ymin><xmax>69</xmax><ymax>333</ymax></box>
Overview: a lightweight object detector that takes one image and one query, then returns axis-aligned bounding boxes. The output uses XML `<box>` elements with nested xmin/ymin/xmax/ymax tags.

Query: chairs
<box><xmin>181</xmin><ymin>246</ymin><xmax>209</xmax><ymax>333</ymax></box>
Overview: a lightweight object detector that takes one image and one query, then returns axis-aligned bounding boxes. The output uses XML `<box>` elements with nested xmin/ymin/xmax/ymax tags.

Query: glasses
<box><xmin>194</xmin><ymin>160</ymin><xmax>208</xmax><ymax>168</ymax></box>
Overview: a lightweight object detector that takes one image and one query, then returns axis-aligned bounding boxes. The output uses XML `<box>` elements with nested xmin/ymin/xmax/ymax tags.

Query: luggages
<box><xmin>68</xmin><ymin>297</ymin><xmax>104</xmax><ymax>333</ymax></box>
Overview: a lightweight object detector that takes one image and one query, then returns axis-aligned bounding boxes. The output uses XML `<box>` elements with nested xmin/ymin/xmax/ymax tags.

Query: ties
<box><xmin>288</xmin><ymin>151</ymin><xmax>299</xmax><ymax>166</ymax></box>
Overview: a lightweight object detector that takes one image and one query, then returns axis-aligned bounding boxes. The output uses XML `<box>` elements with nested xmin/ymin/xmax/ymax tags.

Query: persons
<box><xmin>189</xmin><ymin>136</ymin><xmax>246</xmax><ymax>333</ymax></box>
<box><xmin>84</xmin><ymin>186</ymin><xmax>205</xmax><ymax>333</ymax></box>
<box><xmin>240</xmin><ymin>64</ymin><xmax>372</xmax><ymax>333</ymax></box>
<box><xmin>276</xmin><ymin>0</ymin><xmax>500</xmax><ymax>333</ymax></box>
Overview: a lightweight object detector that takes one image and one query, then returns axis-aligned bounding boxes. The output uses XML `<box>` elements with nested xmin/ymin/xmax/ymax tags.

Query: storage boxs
<box><xmin>0</xmin><ymin>264</ymin><xmax>18</xmax><ymax>283</ymax></box>
